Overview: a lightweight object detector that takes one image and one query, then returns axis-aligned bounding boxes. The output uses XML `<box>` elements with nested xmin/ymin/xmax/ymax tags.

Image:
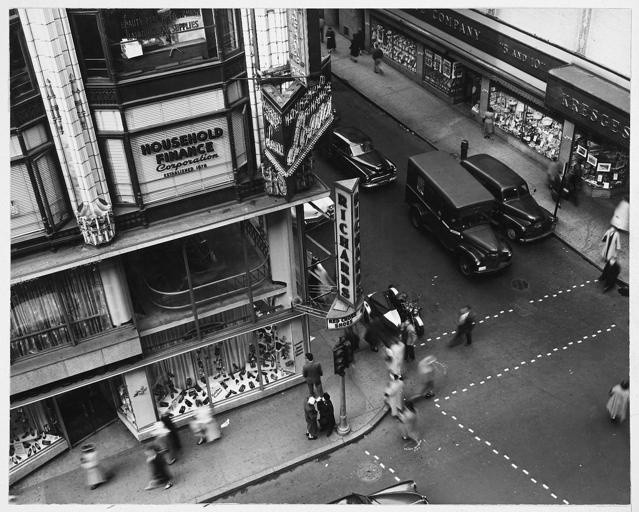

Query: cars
<box><xmin>459</xmin><ymin>152</ymin><xmax>561</xmax><ymax>248</ymax></box>
<box><xmin>401</xmin><ymin>148</ymin><xmax>515</xmax><ymax>279</ymax></box>
<box><xmin>321</xmin><ymin>125</ymin><xmax>399</xmax><ymax>191</ymax></box>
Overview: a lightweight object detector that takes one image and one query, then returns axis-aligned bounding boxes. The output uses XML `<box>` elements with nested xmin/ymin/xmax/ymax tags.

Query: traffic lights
<box><xmin>332</xmin><ymin>334</ymin><xmax>355</xmax><ymax>436</ymax></box>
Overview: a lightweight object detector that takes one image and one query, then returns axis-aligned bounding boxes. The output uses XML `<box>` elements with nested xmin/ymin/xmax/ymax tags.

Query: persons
<box><xmin>446</xmin><ymin>304</ymin><xmax>474</xmax><ymax>348</ymax></box>
<box><xmin>555</xmin><ymin>162</ymin><xmax>579</xmax><ymax>207</ymax></box>
<box><xmin>319</xmin><ymin>16</ymin><xmax>326</xmax><ymax>44</ymax></box>
<box><xmin>325</xmin><ymin>28</ymin><xmax>337</xmax><ymax>55</ymax></box>
<box><xmin>347</xmin><ymin>33</ymin><xmax>361</xmax><ymax>63</ymax></box>
<box><xmin>606</xmin><ymin>377</ymin><xmax>629</xmax><ymax>426</ymax></box>
<box><xmin>355</xmin><ymin>27</ymin><xmax>365</xmax><ymax>54</ymax></box>
<box><xmin>190</xmin><ymin>401</ymin><xmax>221</xmax><ymax>447</ymax></box>
<box><xmin>380</xmin><ymin>318</ymin><xmax>441</xmax><ymax>445</ymax></box>
<box><xmin>599</xmin><ymin>254</ymin><xmax>622</xmax><ymax>294</ymax></box>
<box><xmin>547</xmin><ymin>154</ymin><xmax>562</xmax><ymax>193</ymax></box>
<box><xmin>371</xmin><ymin>44</ymin><xmax>384</xmax><ymax>74</ymax></box>
<box><xmin>79</xmin><ymin>442</ymin><xmax>108</xmax><ymax>490</ymax></box>
<box><xmin>303</xmin><ymin>393</ymin><xmax>320</xmax><ymax>440</ymax></box>
<box><xmin>143</xmin><ymin>446</ymin><xmax>174</xmax><ymax>490</ymax></box>
<box><xmin>302</xmin><ymin>352</ymin><xmax>325</xmax><ymax>397</ymax></box>
<box><xmin>314</xmin><ymin>392</ymin><xmax>337</xmax><ymax>436</ymax></box>
<box><xmin>152</xmin><ymin>416</ymin><xmax>182</xmax><ymax>465</ymax></box>
<box><xmin>597</xmin><ymin>223</ymin><xmax>621</xmax><ymax>266</ymax></box>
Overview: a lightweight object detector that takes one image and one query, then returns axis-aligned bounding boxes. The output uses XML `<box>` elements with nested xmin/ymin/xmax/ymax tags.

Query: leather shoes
<box><xmin>326</xmin><ymin>430</ymin><xmax>332</xmax><ymax>437</ymax></box>
<box><xmin>305</xmin><ymin>432</ymin><xmax>318</xmax><ymax>440</ymax></box>
